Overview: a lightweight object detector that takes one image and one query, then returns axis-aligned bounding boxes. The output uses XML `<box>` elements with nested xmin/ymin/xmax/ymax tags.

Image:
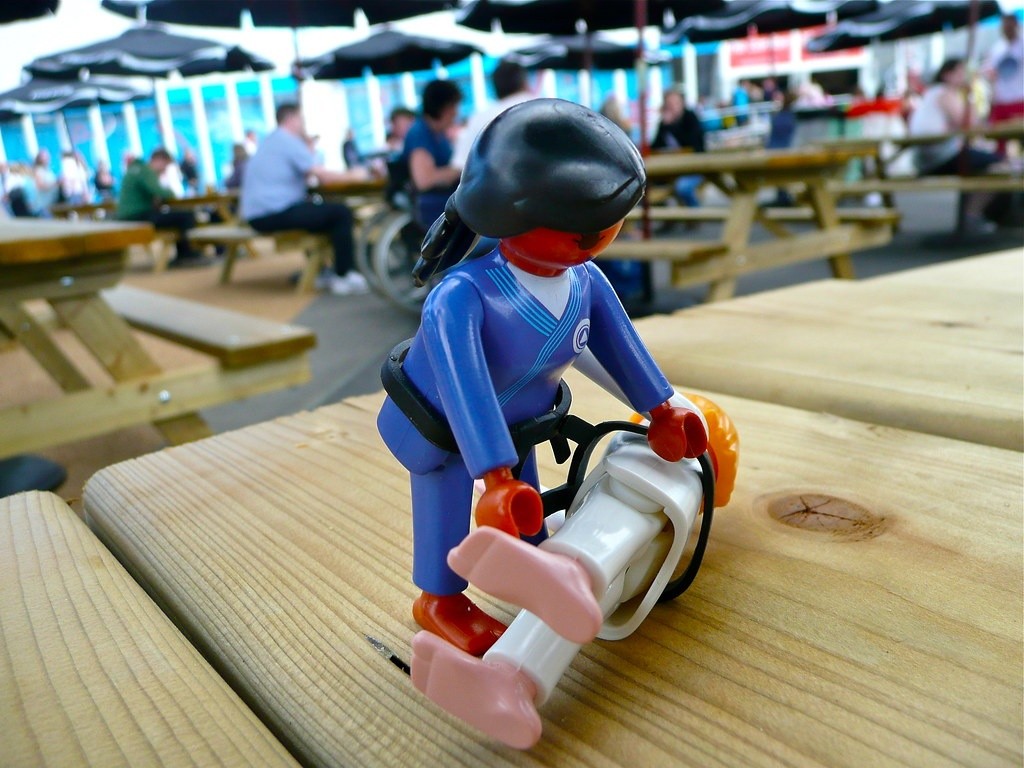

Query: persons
<box><xmin>598</xmin><ymin>87</ymin><xmax>630</xmax><ymax>138</ymax></box>
<box><xmin>411</xmin><ymin>392</ymin><xmax>738</xmax><ymax>749</ymax></box>
<box><xmin>377</xmin><ymin>98</ymin><xmax>709</xmax><ymax>659</ymax></box>
<box><xmin>907</xmin><ymin>57</ymin><xmax>1008</xmax><ymax>239</ymax></box>
<box><xmin>397</xmin><ymin>81</ymin><xmax>501</xmax><ymax>260</ymax></box>
<box><xmin>0</xmin><ymin>131</ymin><xmax>257</xmax><ymax>220</ymax></box>
<box><xmin>238</xmin><ymin>105</ymin><xmax>369</xmax><ymax>294</ymax></box>
<box><xmin>311</xmin><ymin>108</ymin><xmax>416</xmax><ymax>187</ymax></box>
<box><xmin>646</xmin><ymin>91</ymin><xmax>705</xmax><ymax>234</ymax></box>
<box><xmin>985</xmin><ymin>14</ymin><xmax>1024</xmax><ymax>167</ymax></box>
<box><xmin>715</xmin><ymin>76</ymin><xmax>883</xmax><ymax>151</ymax></box>
<box><xmin>451</xmin><ymin>62</ymin><xmax>540</xmax><ymax>185</ymax></box>
<box><xmin>116</xmin><ymin>150</ymin><xmax>207</xmax><ymax>264</ymax></box>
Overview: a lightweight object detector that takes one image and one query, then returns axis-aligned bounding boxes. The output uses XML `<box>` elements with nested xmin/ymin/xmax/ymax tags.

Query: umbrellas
<box><xmin>292</xmin><ymin>31</ymin><xmax>484</xmax><ymax>80</ymax></box>
<box><xmin>807</xmin><ymin>0</ymin><xmax>1001</xmax><ymax>53</ymax></box>
<box><xmin>496</xmin><ymin>36</ymin><xmax>668</xmax><ymax>72</ymax></box>
<box><xmin>23</xmin><ymin>28</ymin><xmax>274</xmax><ymax>80</ymax></box>
<box><xmin>101</xmin><ymin>0</ymin><xmax>462</xmax><ymax>27</ymax></box>
<box><xmin>455</xmin><ymin>0</ymin><xmax>763</xmax><ymax>157</ymax></box>
<box><xmin>0</xmin><ymin>82</ymin><xmax>153</xmax><ymax>125</ymax></box>
<box><xmin>671</xmin><ymin>0</ymin><xmax>893</xmax><ymax>45</ymax></box>
<box><xmin>0</xmin><ymin>0</ymin><xmax>59</xmax><ymax>25</ymax></box>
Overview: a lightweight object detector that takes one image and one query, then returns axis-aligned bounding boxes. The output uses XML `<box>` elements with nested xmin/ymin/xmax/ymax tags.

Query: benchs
<box><xmin>624</xmin><ymin>206</ymin><xmax>905</xmax><ymax>226</ymax></box>
<box><xmin>835</xmin><ymin>176</ymin><xmax>1024</xmax><ymax>191</ymax></box>
<box><xmin>96</xmin><ymin>281</ymin><xmax>319</xmax><ymax>370</ymax></box>
<box><xmin>593</xmin><ymin>237</ymin><xmax>730</xmax><ymax>266</ymax></box>
<box><xmin>183</xmin><ymin>224</ymin><xmax>255</xmax><ymax>243</ymax></box>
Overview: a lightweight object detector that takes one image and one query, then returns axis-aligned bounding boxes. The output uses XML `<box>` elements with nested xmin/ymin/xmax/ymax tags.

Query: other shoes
<box><xmin>329</xmin><ymin>270</ymin><xmax>367</xmax><ymax>295</ymax></box>
<box><xmin>960</xmin><ymin>220</ymin><xmax>996</xmax><ymax>235</ymax></box>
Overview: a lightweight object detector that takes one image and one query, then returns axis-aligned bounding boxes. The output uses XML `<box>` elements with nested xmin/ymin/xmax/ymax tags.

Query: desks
<box><xmin>0</xmin><ymin>116</ymin><xmax>1024</xmax><ymax>768</ymax></box>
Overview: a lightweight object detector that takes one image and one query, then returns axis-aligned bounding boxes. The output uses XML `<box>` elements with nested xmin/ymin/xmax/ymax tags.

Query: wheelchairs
<box><xmin>353</xmin><ymin>151</ymin><xmax>452</xmax><ymax>318</ymax></box>
